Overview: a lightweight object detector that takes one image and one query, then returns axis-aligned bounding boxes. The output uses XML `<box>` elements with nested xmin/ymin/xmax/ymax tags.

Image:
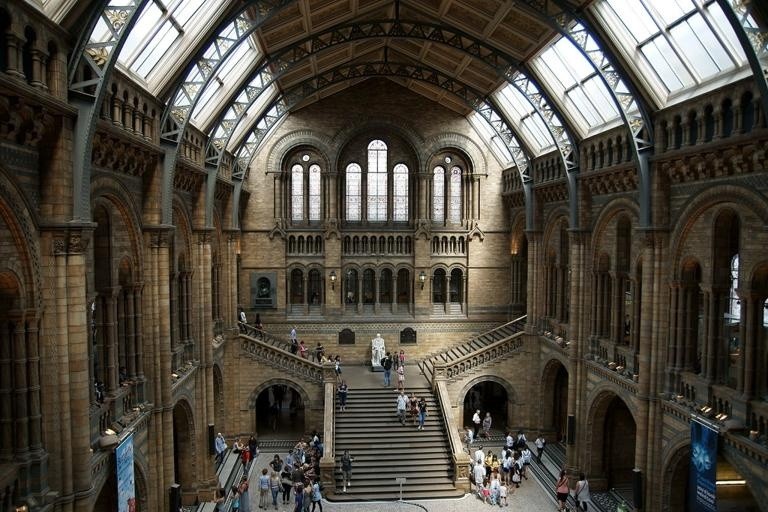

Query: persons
<box><xmin>463</xmin><ymin>397</ymin><xmax>594</xmax><ymax>511</ymax></box>
<box><xmin>211</xmin><ymin>304</ymin><xmax>355</xmax><ymax>512</ymax></box>
<box><xmin>380</xmin><ymin>348</ymin><xmax>426</xmax><ymax>432</ymax></box>
<box><xmin>370</xmin><ymin>331</ymin><xmax>386</xmax><ymax>371</ymax></box>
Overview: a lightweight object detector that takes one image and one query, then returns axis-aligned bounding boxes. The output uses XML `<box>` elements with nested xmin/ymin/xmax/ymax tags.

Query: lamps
<box><xmin>328</xmin><ymin>269</ymin><xmax>339</xmax><ymax>291</ymax></box>
<box><xmin>417</xmin><ymin>270</ymin><xmax>428</xmax><ymax>291</ymax></box>
